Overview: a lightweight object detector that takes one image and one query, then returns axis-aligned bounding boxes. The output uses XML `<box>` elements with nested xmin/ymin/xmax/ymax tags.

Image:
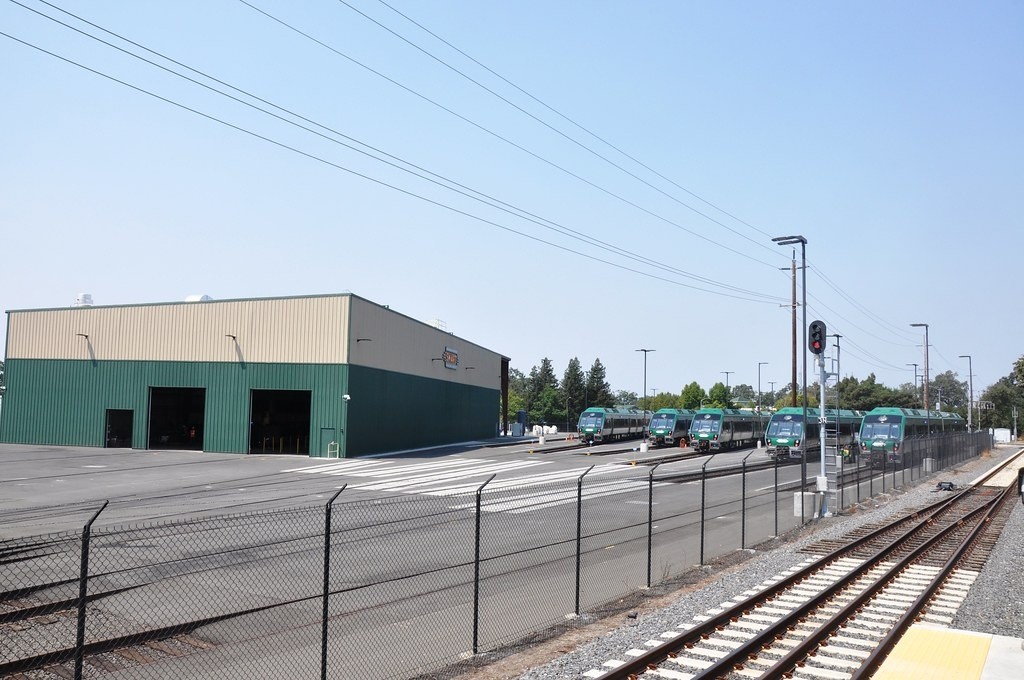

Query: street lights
<box><xmin>772</xmin><ymin>234</ymin><xmax>809</xmax><ymax>492</ymax></box>
<box><xmin>768</xmin><ymin>382</ymin><xmax>777</xmax><ymax>398</ymax></box>
<box><xmin>635</xmin><ymin>349</ymin><xmax>655</xmax><ymax>443</ymax></box>
<box><xmin>757</xmin><ymin>363</ymin><xmax>769</xmax><ymax>408</ymax></box>
<box><xmin>906</xmin><ymin>323</ymin><xmax>973</xmax><ymax>436</ymax></box>
<box><xmin>720</xmin><ymin>371</ymin><xmax>735</xmax><ymax>408</ymax></box>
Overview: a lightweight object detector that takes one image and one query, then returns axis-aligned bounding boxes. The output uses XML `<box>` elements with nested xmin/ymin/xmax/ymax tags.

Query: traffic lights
<box><xmin>808</xmin><ymin>319</ymin><xmax>828</xmax><ymax>356</ymax></box>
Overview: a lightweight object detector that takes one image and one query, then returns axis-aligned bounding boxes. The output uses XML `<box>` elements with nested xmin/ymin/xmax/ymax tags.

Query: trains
<box><xmin>859</xmin><ymin>407</ymin><xmax>965</xmax><ymax>469</ymax></box>
<box><xmin>576</xmin><ymin>405</ymin><xmax>656</xmax><ymax>445</ymax></box>
<box><xmin>688</xmin><ymin>407</ymin><xmax>779</xmax><ymax>453</ymax></box>
<box><xmin>648</xmin><ymin>409</ymin><xmax>701</xmax><ymax>446</ymax></box>
<box><xmin>765</xmin><ymin>407</ymin><xmax>870</xmax><ymax>463</ymax></box>
